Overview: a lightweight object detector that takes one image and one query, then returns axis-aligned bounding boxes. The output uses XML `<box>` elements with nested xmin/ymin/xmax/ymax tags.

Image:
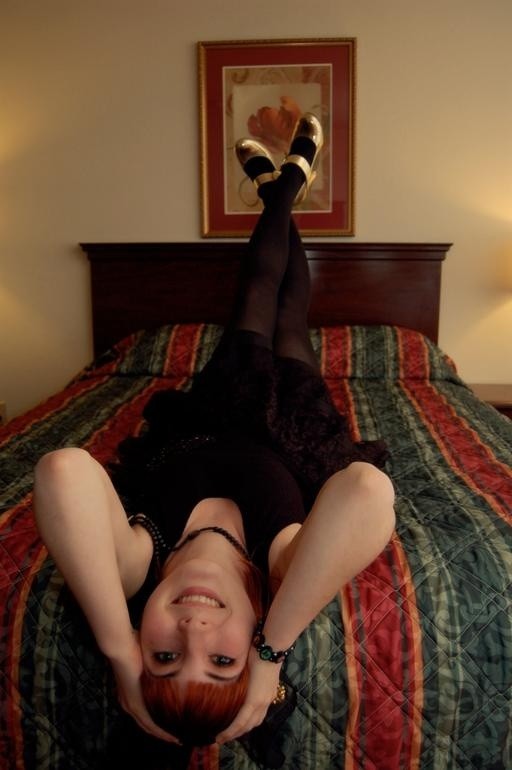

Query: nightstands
<box><xmin>466</xmin><ymin>383</ymin><xmax>512</xmax><ymax>421</ymax></box>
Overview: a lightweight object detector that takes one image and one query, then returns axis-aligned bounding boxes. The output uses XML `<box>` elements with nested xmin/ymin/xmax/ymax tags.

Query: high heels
<box><xmin>233</xmin><ymin>137</ymin><xmax>280</xmax><ymax>197</ymax></box>
<box><xmin>281</xmin><ymin>112</ymin><xmax>324</xmax><ymax>205</ymax></box>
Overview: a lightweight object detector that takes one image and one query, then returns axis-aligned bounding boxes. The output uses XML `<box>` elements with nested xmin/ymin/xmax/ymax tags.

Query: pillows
<box><xmin>78</xmin><ymin>326</ymin><xmax>471</xmax><ymax>391</ymax></box>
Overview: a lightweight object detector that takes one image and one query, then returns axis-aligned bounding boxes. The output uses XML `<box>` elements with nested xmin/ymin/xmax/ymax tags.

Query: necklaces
<box><xmin>131</xmin><ymin>510</ymin><xmax>251</xmax><ymax>577</ymax></box>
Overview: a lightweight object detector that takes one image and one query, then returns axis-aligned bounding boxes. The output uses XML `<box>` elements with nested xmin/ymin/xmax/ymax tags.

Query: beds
<box><xmin>0</xmin><ymin>242</ymin><xmax>512</xmax><ymax>770</ymax></box>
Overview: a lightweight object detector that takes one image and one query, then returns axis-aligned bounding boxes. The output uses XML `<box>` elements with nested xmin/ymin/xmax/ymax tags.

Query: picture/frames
<box><xmin>196</xmin><ymin>37</ymin><xmax>357</xmax><ymax>238</ymax></box>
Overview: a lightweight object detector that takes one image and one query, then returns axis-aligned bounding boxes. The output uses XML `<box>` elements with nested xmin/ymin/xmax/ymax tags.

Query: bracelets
<box><xmin>251</xmin><ymin>614</ymin><xmax>297</xmax><ymax>663</ymax></box>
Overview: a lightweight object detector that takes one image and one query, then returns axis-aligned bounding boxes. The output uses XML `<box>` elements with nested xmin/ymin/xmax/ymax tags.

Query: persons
<box><xmin>32</xmin><ymin>111</ymin><xmax>397</xmax><ymax>746</ymax></box>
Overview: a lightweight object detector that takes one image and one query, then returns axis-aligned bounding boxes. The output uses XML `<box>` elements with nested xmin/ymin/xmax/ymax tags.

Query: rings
<box><xmin>274</xmin><ymin>683</ymin><xmax>286</xmax><ymax>706</ymax></box>
